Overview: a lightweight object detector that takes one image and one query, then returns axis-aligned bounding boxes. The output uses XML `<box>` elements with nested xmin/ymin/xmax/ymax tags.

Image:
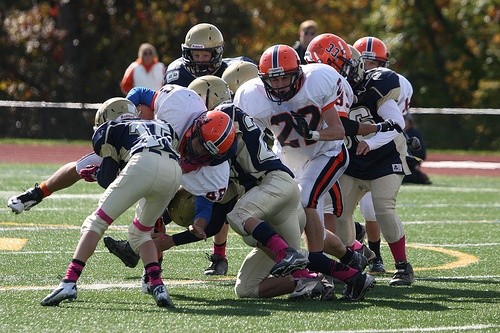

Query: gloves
<box><xmin>77</xmin><ymin>164</ymin><xmax>101</xmax><ymax>182</ymax></box>
<box><xmin>377</xmin><ymin>118</ymin><xmax>403</xmax><ymax>133</ymax></box>
<box><xmin>288</xmin><ymin>111</ymin><xmax>320</xmax><ymax>140</ymax></box>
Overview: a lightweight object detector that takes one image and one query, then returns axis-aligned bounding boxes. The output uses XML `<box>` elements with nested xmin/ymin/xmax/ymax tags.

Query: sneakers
<box><xmin>104</xmin><ymin>236</ymin><xmax>141</xmax><ymax>268</ymax></box>
<box><xmin>204</xmin><ymin>252</ymin><xmax>228</xmax><ymax>275</ymax></box>
<box><xmin>354</xmin><ymin>221</ymin><xmax>366</xmax><ymax>242</ymax></box>
<box><xmin>270</xmin><ymin>247</ymin><xmax>310</xmax><ymax>277</ymax></box>
<box><xmin>141</xmin><ymin>268</ymin><xmax>152</xmax><ymax>293</ymax></box>
<box><xmin>40</xmin><ymin>279</ymin><xmax>77</xmax><ymax>305</ymax></box>
<box><xmin>152</xmin><ymin>282</ymin><xmax>174</xmax><ymax>307</ymax></box>
<box><xmin>369</xmin><ymin>257</ymin><xmax>385</xmax><ymax>273</ymax></box>
<box><xmin>288</xmin><ymin>243</ymin><xmax>377</xmax><ymax>302</ymax></box>
<box><xmin>389</xmin><ymin>258</ymin><xmax>414</xmax><ymax>286</ymax></box>
<box><xmin>7</xmin><ymin>182</ymin><xmax>45</xmax><ymax>214</ymax></box>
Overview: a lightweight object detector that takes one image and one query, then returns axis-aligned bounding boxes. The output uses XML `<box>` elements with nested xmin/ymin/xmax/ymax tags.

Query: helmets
<box><xmin>257</xmin><ymin>45</ymin><xmax>303</xmax><ymax>103</ymax></box>
<box><xmin>190</xmin><ymin>74</ymin><xmax>235</xmax><ymax>110</ymax></box>
<box><xmin>178</xmin><ymin>109</ymin><xmax>236</xmax><ymax>173</ymax></box>
<box><xmin>353</xmin><ymin>36</ymin><xmax>390</xmax><ymax>68</ymax></box>
<box><xmin>340</xmin><ymin>44</ymin><xmax>365</xmax><ymax>89</ymax></box>
<box><xmin>168</xmin><ymin>186</ymin><xmax>196</xmax><ymax>228</ymax></box>
<box><xmin>180</xmin><ymin>23</ymin><xmax>225</xmax><ymax>78</ymax></box>
<box><xmin>304</xmin><ymin>32</ymin><xmax>353</xmax><ymax>72</ymax></box>
<box><xmin>93</xmin><ymin>97</ymin><xmax>141</xmax><ymax>132</ymax></box>
<box><xmin>221</xmin><ymin>60</ymin><xmax>260</xmax><ymax>96</ymax></box>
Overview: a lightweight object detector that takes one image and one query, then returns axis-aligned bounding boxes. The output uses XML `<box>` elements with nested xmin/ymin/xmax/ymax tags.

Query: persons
<box><xmin>8</xmin><ymin>20</ymin><xmax>426</xmax><ymax>299</ymax></box>
<box><xmin>39</xmin><ymin>99</ymin><xmax>183</xmax><ymax>308</ymax></box>
